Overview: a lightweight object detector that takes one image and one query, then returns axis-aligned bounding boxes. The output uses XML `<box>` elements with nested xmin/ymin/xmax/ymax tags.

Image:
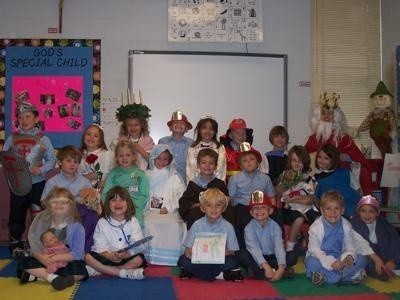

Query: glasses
<box><xmin>291</xmin><ymin>158</ymin><xmax>302</xmax><ymax>164</ymax></box>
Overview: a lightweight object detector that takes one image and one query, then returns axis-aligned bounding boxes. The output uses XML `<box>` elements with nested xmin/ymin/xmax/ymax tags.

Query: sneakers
<box><xmin>126</xmin><ymin>267</ymin><xmax>145</xmax><ymax>280</ymax></box>
<box><xmin>51</xmin><ymin>274</ymin><xmax>75</xmax><ymax>290</ymax></box>
<box><xmin>351</xmin><ymin>268</ymin><xmax>367</xmax><ymax>285</ymax></box>
<box><xmin>20</xmin><ymin>271</ymin><xmax>37</xmax><ymax>284</ymax></box>
<box><xmin>223</xmin><ymin>268</ymin><xmax>243</xmax><ymax>281</ymax></box>
<box><xmin>274</xmin><ymin>265</ymin><xmax>294</xmax><ymax>279</ymax></box>
<box><xmin>85</xmin><ymin>265</ymin><xmax>103</xmax><ymax>277</ymax></box>
<box><xmin>311</xmin><ymin>272</ymin><xmax>324</xmax><ymax>286</ymax></box>
<box><xmin>367</xmin><ymin>265</ymin><xmax>389</xmax><ymax>281</ymax></box>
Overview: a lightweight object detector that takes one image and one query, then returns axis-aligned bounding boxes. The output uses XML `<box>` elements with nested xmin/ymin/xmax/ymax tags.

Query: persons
<box><xmin>2</xmin><ymin>89</ymin><xmax>399</xmax><ymax>291</ymax></box>
<box><xmin>358</xmin><ymin>82</ymin><xmax>397</xmax><ymax>159</ymax></box>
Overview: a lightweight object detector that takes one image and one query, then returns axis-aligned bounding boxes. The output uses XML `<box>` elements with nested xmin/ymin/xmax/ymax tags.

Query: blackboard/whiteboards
<box><xmin>128</xmin><ymin>50</ymin><xmax>287</xmax><ymax>155</ymax></box>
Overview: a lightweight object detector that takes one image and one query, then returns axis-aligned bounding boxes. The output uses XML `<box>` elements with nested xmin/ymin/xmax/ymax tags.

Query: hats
<box><xmin>320</xmin><ymin>91</ymin><xmax>341</xmax><ymax>110</ymax></box>
<box><xmin>16</xmin><ymin>101</ymin><xmax>37</xmax><ymax>113</ymax></box>
<box><xmin>167</xmin><ymin>111</ymin><xmax>192</xmax><ymax>129</ymax></box>
<box><xmin>116</xmin><ymin>88</ymin><xmax>151</xmax><ymax>121</ymax></box>
<box><xmin>249</xmin><ymin>188</ymin><xmax>272</xmax><ymax>209</ymax></box>
<box><xmin>229</xmin><ymin>119</ymin><xmax>246</xmax><ymax>129</ymax></box>
<box><xmin>234</xmin><ymin>141</ymin><xmax>262</xmax><ymax>164</ymax></box>
<box><xmin>358</xmin><ymin>195</ymin><xmax>378</xmax><ymax>206</ymax></box>
